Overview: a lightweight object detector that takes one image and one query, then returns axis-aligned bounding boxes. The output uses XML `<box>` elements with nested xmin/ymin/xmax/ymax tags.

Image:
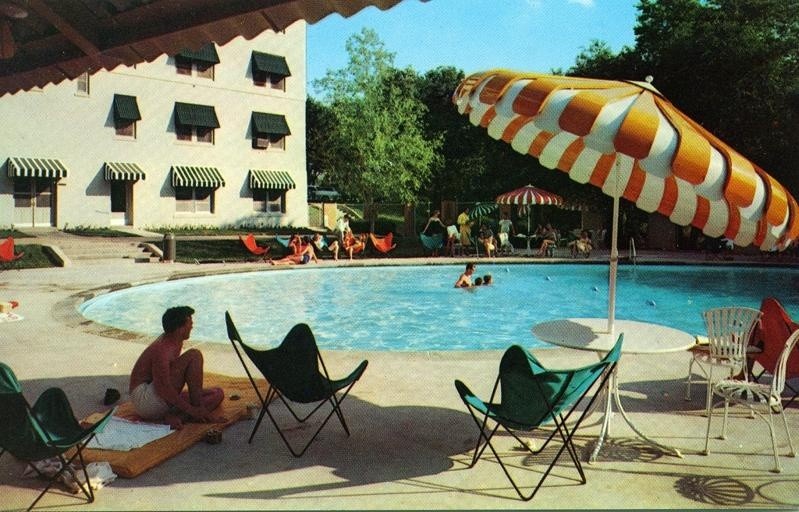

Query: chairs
<box><xmin>685</xmin><ymin>297</ymin><xmax>799</xmax><ymax>472</ymax></box>
<box><xmin>1</xmin><ymin>236</ymin><xmax>26</xmax><ymax>273</ymax></box>
<box><xmin>452</xmin><ymin>330</ymin><xmax>626</xmax><ymax>502</ymax></box>
<box><xmin>222</xmin><ymin>310</ymin><xmax>370</xmax><ymax>459</ymax></box>
<box><xmin>0</xmin><ymin>363</ymin><xmax>117</xmax><ymax>512</ymax></box>
<box><xmin>236</xmin><ymin>230</ymin><xmax>397</xmax><ymax>264</ymax></box>
<box><xmin>419</xmin><ymin>232</ymin><xmax>592</xmax><ymax>259</ymax></box>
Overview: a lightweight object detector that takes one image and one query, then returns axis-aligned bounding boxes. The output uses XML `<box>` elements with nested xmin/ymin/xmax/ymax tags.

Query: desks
<box><xmin>530</xmin><ymin>316</ymin><xmax>698</xmax><ymax>464</ymax></box>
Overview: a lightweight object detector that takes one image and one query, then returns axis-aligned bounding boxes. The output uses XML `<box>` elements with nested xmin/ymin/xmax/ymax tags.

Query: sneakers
<box><xmin>104</xmin><ymin>388</ymin><xmax>120</xmax><ymax>405</ymax></box>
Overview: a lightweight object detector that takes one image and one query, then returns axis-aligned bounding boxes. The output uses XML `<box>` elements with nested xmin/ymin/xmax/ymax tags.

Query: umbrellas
<box><xmin>452</xmin><ymin>65</ymin><xmax>799</xmax><ymax>333</ymax></box>
<box><xmin>470</xmin><ymin>197</ymin><xmax>498</xmax><ymax>220</ymax></box>
<box><xmin>495</xmin><ymin>182</ymin><xmax>562</xmax><ymax>251</ymax></box>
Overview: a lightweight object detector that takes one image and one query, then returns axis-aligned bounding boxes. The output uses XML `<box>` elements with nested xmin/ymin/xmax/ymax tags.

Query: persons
<box><xmin>475</xmin><ymin>277</ymin><xmax>483</xmax><ymax>287</ymax></box>
<box><xmin>267</xmin><ymin>212</ymin><xmax>367</xmax><ymax>266</ymax></box>
<box><xmin>128</xmin><ymin>305</ymin><xmax>228</xmax><ymax>431</ymax></box>
<box><xmin>454</xmin><ymin>262</ymin><xmax>477</xmax><ymax>290</ymax></box>
<box><xmin>422</xmin><ymin>208</ymin><xmax>593</xmax><ymax>259</ymax></box>
<box><xmin>482</xmin><ymin>274</ymin><xmax>493</xmax><ymax>285</ymax></box>
